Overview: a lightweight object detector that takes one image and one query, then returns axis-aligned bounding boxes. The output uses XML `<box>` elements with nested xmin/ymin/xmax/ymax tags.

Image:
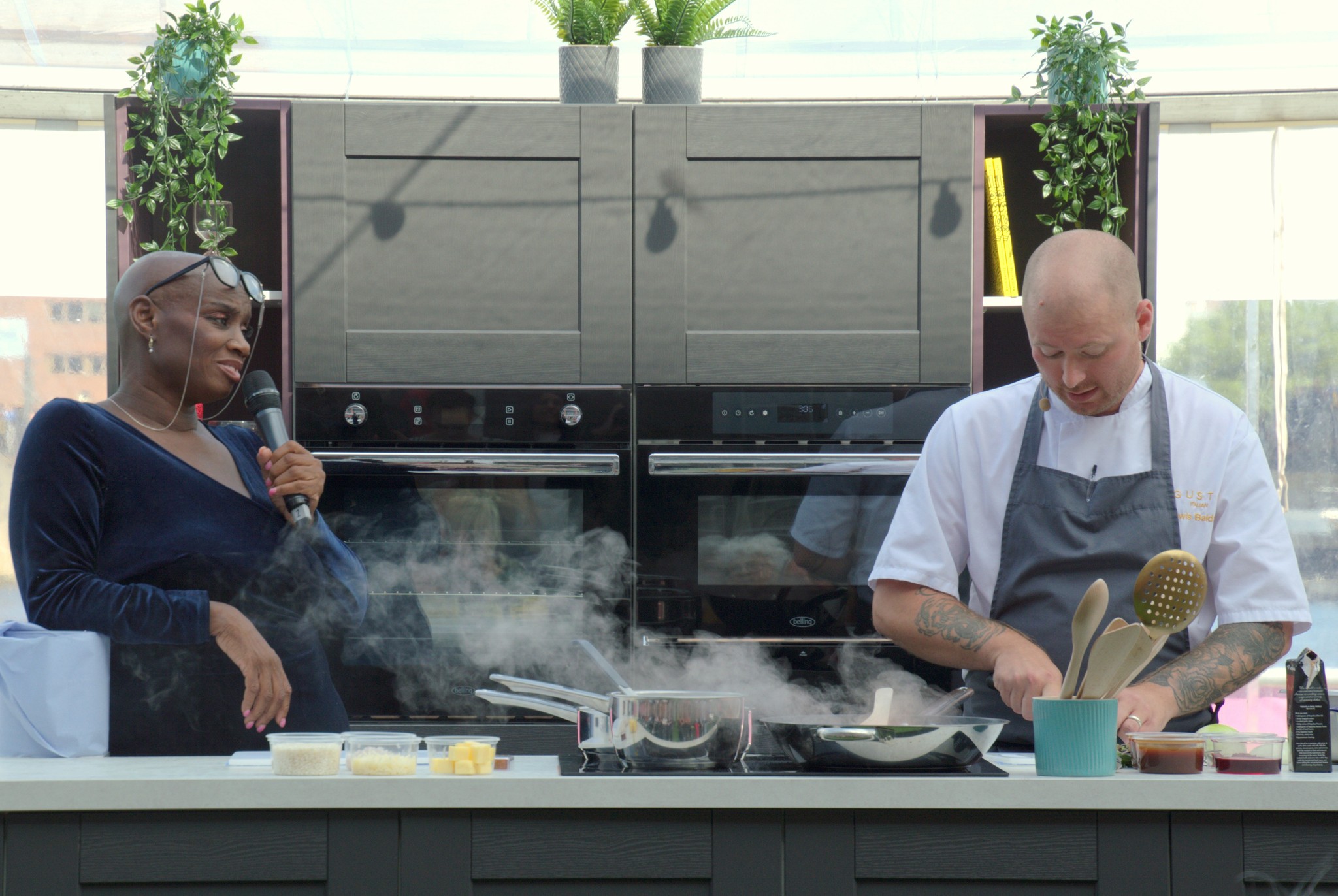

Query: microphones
<box><xmin>1039</xmin><ymin>374</ymin><xmax>1050</xmax><ymax>412</ymax></box>
<box><xmin>241</xmin><ymin>370</ymin><xmax>312</xmax><ymax>531</ymax></box>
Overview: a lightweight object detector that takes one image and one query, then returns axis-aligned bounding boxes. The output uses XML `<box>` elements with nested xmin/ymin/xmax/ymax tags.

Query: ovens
<box><xmin>291</xmin><ymin>382</ymin><xmax>968</xmax><ymax>723</ymax></box>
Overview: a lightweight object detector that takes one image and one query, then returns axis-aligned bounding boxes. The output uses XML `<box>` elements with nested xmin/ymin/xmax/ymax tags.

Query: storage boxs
<box><xmin>1285</xmin><ymin>648</ymin><xmax>1334</xmax><ymax>772</ymax></box>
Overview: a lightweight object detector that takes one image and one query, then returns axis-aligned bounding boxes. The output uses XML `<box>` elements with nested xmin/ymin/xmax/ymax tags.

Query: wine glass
<box><xmin>193</xmin><ymin>200</ymin><xmax>232</xmax><ymax>256</ymax></box>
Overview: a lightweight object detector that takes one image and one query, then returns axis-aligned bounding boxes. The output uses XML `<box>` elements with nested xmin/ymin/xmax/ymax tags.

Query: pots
<box><xmin>756</xmin><ymin>685</ymin><xmax>1011</xmax><ymax>770</ymax></box>
<box><xmin>473</xmin><ymin>674</ymin><xmax>755</xmax><ymax>769</ymax></box>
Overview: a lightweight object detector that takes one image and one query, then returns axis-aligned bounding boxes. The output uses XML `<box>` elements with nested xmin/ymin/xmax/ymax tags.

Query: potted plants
<box><xmin>629</xmin><ymin>0</ymin><xmax>777</xmax><ymax>105</ymax></box>
<box><xmin>106</xmin><ymin>0</ymin><xmax>261</xmax><ymax>262</ymax></box>
<box><xmin>530</xmin><ymin>0</ymin><xmax>643</xmax><ymax>104</ymax></box>
<box><xmin>1002</xmin><ymin>10</ymin><xmax>1152</xmax><ymax>246</ymax></box>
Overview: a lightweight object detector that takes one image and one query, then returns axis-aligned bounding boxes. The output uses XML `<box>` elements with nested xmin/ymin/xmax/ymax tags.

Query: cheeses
<box><xmin>429</xmin><ymin>740</ymin><xmax>497</xmax><ymax>775</ymax></box>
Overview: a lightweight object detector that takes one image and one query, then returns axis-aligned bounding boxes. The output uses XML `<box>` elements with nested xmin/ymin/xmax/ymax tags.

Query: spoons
<box><xmin>1058</xmin><ymin>577</ymin><xmax>1110</xmax><ymax>699</ymax></box>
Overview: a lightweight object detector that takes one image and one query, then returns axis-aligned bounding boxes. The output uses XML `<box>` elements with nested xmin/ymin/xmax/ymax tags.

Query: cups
<box><xmin>1031</xmin><ymin>695</ymin><xmax>1119</xmax><ymax>777</ymax></box>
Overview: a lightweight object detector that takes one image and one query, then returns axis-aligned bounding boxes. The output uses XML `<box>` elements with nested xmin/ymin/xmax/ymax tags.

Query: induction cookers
<box><xmin>578</xmin><ymin>755</ymin><xmax>1010</xmax><ymax>777</ymax></box>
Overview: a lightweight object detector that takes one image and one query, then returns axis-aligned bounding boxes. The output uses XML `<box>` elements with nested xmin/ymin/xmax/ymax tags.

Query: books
<box><xmin>984</xmin><ymin>157</ymin><xmax>1012</xmax><ymax>297</ymax></box>
<box><xmin>994</xmin><ymin>157</ymin><xmax>1018</xmax><ymax>297</ymax></box>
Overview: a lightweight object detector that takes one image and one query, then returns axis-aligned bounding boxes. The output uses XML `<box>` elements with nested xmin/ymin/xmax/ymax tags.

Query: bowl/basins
<box><xmin>1126</xmin><ymin>730</ymin><xmax>1286</xmax><ymax>775</ymax></box>
<box><xmin>267</xmin><ymin>730</ymin><xmax>500</xmax><ymax>777</ymax></box>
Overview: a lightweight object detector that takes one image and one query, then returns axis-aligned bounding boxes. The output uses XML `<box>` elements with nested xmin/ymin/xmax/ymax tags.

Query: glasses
<box><xmin>143</xmin><ymin>255</ymin><xmax>266</xmax><ymax>304</ymax></box>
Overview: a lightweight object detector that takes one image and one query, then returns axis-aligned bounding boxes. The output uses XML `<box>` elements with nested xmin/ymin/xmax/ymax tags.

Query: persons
<box><xmin>10</xmin><ymin>249</ymin><xmax>368</xmax><ymax>756</ymax></box>
<box><xmin>790</xmin><ymin>389</ymin><xmax>961</xmax><ymax>644</ymax></box>
<box><xmin>867</xmin><ymin>227</ymin><xmax>1311</xmax><ymax>744</ymax></box>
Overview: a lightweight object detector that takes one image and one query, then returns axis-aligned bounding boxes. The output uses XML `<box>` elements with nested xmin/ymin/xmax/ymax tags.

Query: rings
<box><xmin>1128</xmin><ymin>716</ymin><xmax>1142</xmax><ymax>726</ymax></box>
